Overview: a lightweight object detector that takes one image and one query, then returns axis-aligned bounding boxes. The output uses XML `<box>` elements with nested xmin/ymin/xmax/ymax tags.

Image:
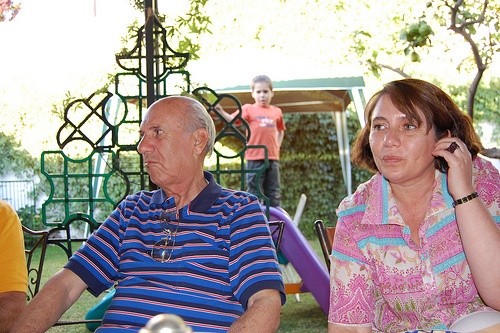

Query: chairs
<box><xmin>21</xmin><ymin>224</ymin><xmax>49</xmax><ymax>301</ymax></box>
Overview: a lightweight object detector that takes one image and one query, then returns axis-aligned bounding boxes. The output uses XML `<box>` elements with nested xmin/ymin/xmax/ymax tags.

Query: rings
<box><xmin>449</xmin><ymin>142</ymin><xmax>458</xmax><ymax>152</ymax></box>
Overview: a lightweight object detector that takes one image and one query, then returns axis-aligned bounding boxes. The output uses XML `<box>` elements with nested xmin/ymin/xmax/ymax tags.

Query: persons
<box><xmin>328</xmin><ymin>79</ymin><xmax>500</xmax><ymax>333</ymax></box>
<box><xmin>12</xmin><ymin>96</ymin><xmax>286</xmax><ymax>333</ymax></box>
<box><xmin>0</xmin><ymin>200</ymin><xmax>29</xmax><ymax>333</ymax></box>
<box><xmin>215</xmin><ymin>76</ymin><xmax>286</xmax><ymax>207</ymax></box>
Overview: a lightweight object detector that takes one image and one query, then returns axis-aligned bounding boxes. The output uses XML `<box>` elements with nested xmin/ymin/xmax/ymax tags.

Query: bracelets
<box><xmin>455</xmin><ymin>192</ymin><xmax>479</xmax><ymax>205</ymax></box>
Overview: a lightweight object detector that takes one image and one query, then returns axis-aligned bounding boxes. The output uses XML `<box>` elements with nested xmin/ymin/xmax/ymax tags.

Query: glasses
<box><xmin>146</xmin><ymin>207</ymin><xmax>181</xmax><ymax>263</ymax></box>
